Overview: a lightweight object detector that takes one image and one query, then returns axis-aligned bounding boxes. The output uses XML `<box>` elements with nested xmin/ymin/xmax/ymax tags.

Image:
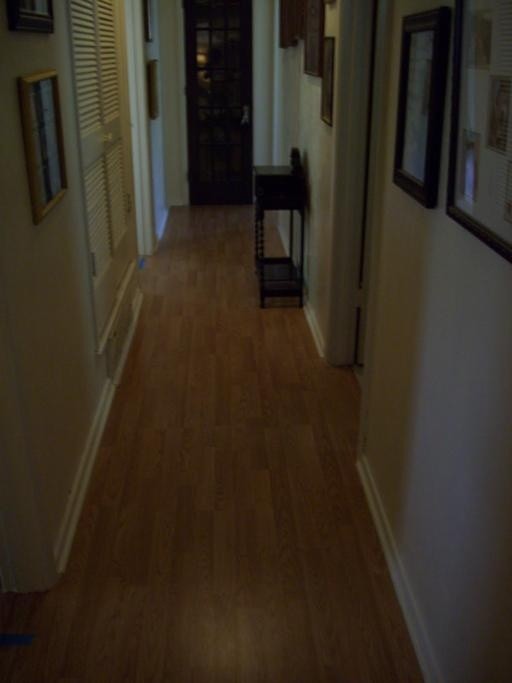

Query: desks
<box><xmin>252</xmin><ymin>148</ymin><xmax>305</xmax><ymax>309</ymax></box>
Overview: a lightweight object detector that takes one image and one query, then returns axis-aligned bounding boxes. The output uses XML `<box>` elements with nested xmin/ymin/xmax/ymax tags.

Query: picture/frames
<box><xmin>17</xmin><ymin>69</ymin><xmax>68</xmax><ymax>225</ymax></box>
<box><xmin>304</xmin><ymin>0</ymin><xmax>324</xmax><ymax>77</ymax></box>
<box><xmin>393</xmin><ymin>6</ymin><xmax>452</xmax><ymax>209</ymax></box>
<box><xmin>447</xmin><ymin>0</ymin><xmax>512</xmax><ymax>263</ymax></box>
<box><xmin>321</xmin><ymin>37</ymin><xmax>334</xmax><ymax>127</ymax></box>
<box><xmin>6</xmin><ymin>0</ymin><xmax>53</xmax><ymax>33</ymax></box>
